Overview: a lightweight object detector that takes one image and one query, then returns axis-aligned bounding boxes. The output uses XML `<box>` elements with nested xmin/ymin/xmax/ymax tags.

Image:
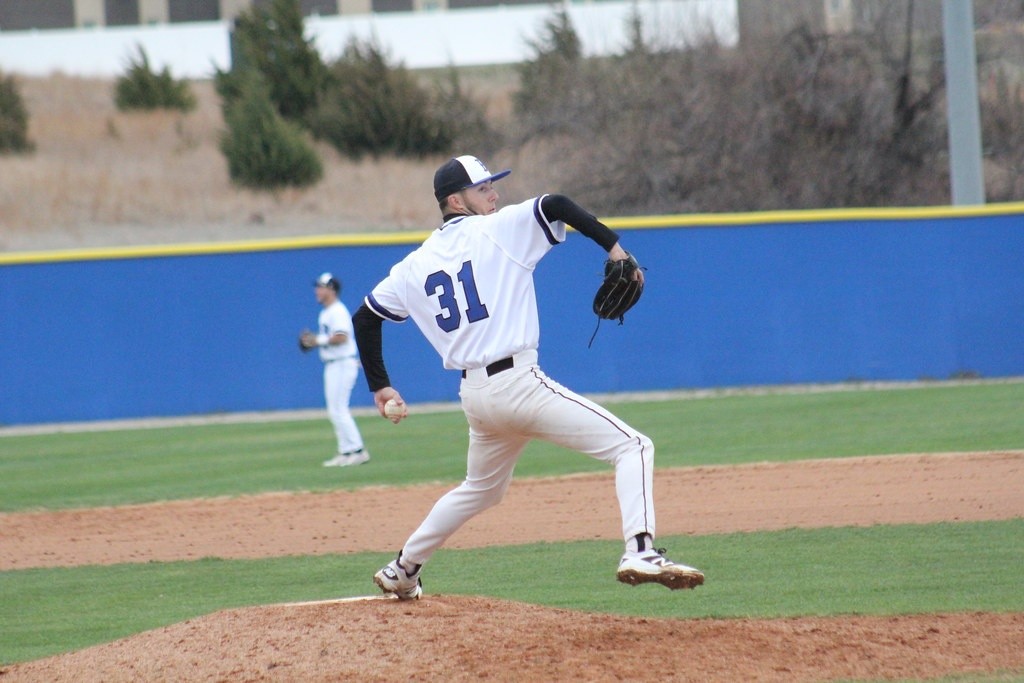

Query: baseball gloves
<box><xmin>300</xmin><ymin>331</ymin><xmax>315</xmax><ymax>354</ymax></box>
<box><xmin>587</xmin><ymin>250</ymin><xmax>649</xmax><ymax>349</ymax></box>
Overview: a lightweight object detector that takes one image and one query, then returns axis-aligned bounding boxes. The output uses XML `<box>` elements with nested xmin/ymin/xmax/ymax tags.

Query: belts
<box><xmin>461</xmin><ymin>357</ymin><xmax>514</xmax><ymax>378</ymax></box>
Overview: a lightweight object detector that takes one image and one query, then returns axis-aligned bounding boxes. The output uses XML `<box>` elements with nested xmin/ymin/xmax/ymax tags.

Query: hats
<box><xmin>312</xmin><ymin>271</ymin><xmax>340</xmax><ymax>290</ymax></box>
<box><xmin>434</xmin><ymin>155</ymin><xmax>511</xmax><ymax>202</ymax></box>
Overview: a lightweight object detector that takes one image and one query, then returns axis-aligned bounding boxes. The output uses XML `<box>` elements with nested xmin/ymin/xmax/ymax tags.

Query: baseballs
<box><xmin>385</xmin><ymin>398</ymin><xmax>406</xmax><ymax>418</ymax></box>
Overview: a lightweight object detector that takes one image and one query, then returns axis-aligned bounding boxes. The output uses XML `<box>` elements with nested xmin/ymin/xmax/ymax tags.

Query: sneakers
<box><xmin>616</xmin><ymin>548</ymin><xmax>704</xmax><ymax>590</ymax></box>
<box><xmin>374</xmin><ymin>548</ymin><xmax>423</xmax><ymax>600</ymax></box>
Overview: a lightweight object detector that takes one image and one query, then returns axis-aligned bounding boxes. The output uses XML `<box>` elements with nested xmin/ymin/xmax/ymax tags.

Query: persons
<box><xmin>351</xmin><ymin>155</ymin><xmax>705</xmax><ymax>599</ymax></box>
<box><xmin>301</xmin><ymin>272</ymin><xmax>371</xmax><ymax>468</ymax></box>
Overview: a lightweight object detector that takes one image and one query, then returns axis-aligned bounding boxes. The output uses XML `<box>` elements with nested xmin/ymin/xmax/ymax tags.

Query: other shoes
<box><xmin>323</xmin><ymin>450</ymin><xmax>370</xmax><ymax>469</ymax></box>
